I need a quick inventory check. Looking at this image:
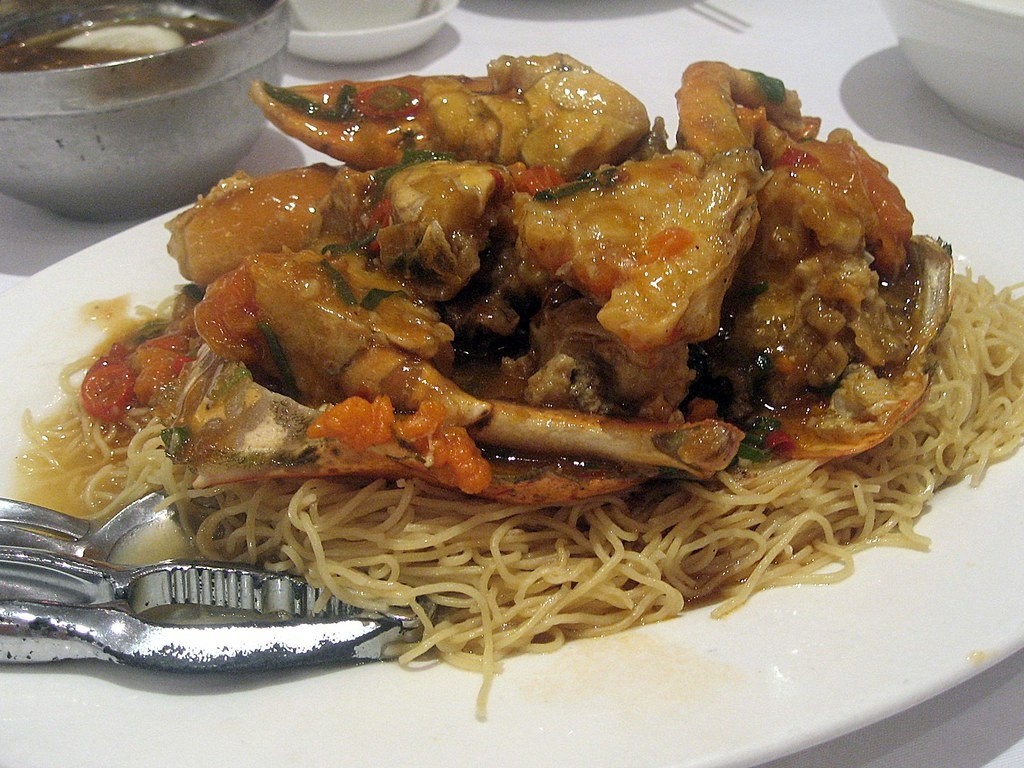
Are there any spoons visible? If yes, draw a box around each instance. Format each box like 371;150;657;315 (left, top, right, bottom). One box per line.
0;489;268;567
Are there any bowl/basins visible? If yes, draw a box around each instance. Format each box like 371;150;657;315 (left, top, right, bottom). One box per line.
0;1;290;223
885;0;1024;147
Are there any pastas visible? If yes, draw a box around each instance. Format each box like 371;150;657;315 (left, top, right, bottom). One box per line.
18;254;1024;719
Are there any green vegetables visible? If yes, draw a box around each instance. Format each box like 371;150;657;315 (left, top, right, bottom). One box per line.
249;67;788;397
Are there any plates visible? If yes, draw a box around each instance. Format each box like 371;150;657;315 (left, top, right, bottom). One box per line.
3;146;1023;767
282;1;459;63
